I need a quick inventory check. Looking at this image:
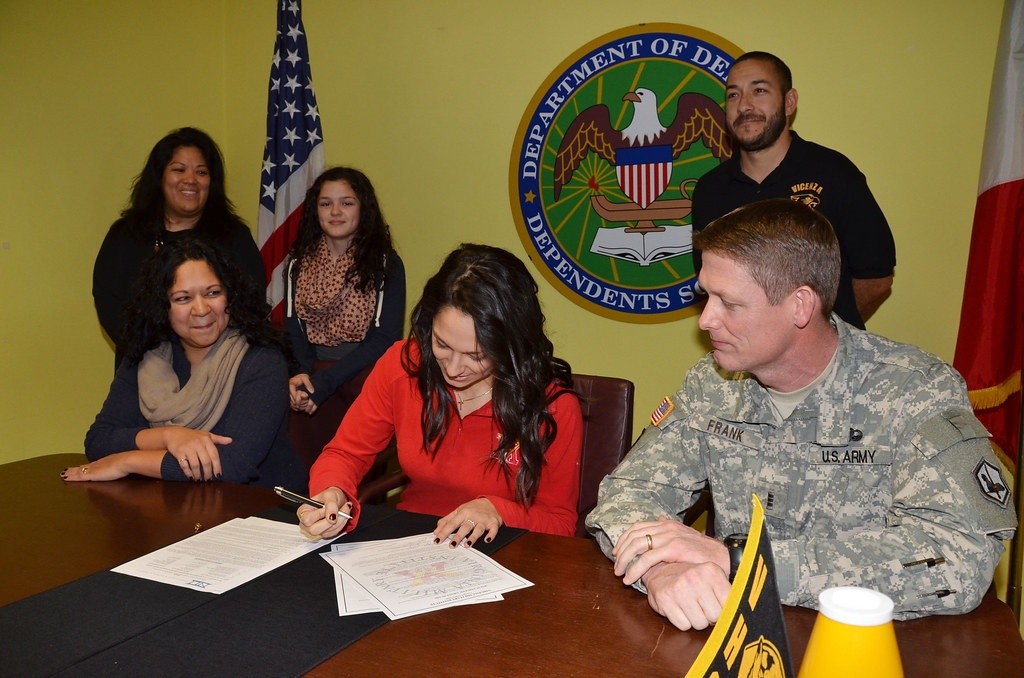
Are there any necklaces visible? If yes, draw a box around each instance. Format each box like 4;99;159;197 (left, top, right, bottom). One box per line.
457;387;493;411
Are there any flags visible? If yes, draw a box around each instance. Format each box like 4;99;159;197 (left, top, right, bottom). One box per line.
257;0;326;332
952;0;1024;470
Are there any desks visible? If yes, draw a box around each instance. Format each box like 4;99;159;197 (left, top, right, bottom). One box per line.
0;451;1024;678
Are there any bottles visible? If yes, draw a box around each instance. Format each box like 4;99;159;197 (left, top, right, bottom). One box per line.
798;586;904;678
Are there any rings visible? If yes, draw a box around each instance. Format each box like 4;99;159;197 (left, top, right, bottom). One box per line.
179;459;187;464
468;520;475;526
299;511;306;518
646;534;652;550
82;467;88;474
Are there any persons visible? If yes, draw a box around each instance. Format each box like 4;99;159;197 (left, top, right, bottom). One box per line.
691;51;897;331
59;237;307;494
282;166;406;415
296;243;583;549
584;199;1019;631
92;127;273;374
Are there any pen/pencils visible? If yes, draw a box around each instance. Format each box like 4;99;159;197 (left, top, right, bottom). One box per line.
916;588;958;599
904;556;945;568
274;485;354;520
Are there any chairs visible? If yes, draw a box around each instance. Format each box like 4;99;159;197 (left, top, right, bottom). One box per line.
558;374;634;537
283;359;411;512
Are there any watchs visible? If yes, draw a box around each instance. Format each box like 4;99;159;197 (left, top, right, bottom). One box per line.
723;533;748;585
